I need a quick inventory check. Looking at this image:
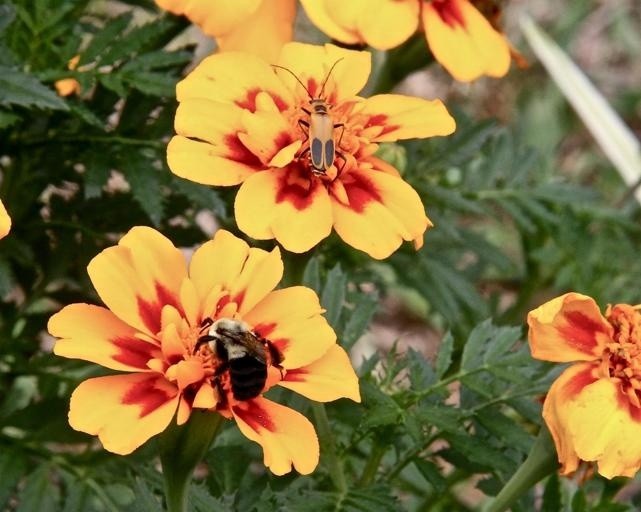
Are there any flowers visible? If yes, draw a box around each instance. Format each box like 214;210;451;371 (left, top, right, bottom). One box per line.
1;0;641;512
